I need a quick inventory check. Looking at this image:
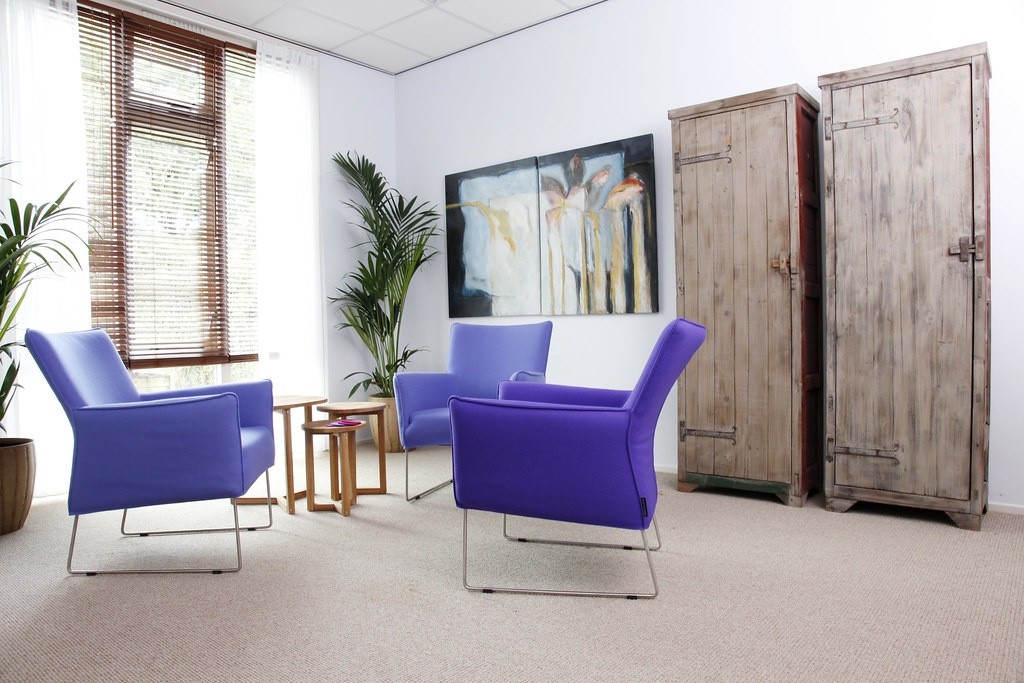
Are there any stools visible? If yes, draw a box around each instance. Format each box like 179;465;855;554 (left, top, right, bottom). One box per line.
302;418;366;517
316;401;385;495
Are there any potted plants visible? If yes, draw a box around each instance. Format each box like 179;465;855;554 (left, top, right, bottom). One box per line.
331;150;449;452
0;161;106;535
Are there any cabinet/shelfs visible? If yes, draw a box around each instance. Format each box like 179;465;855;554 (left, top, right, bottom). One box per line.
816;41;993;531
667;84;822;507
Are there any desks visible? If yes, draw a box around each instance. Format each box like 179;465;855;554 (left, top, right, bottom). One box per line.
230;397;327;515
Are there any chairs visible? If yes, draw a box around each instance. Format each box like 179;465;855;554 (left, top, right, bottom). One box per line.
448;319;706;599
24;327;276;575
393;320;552;501
